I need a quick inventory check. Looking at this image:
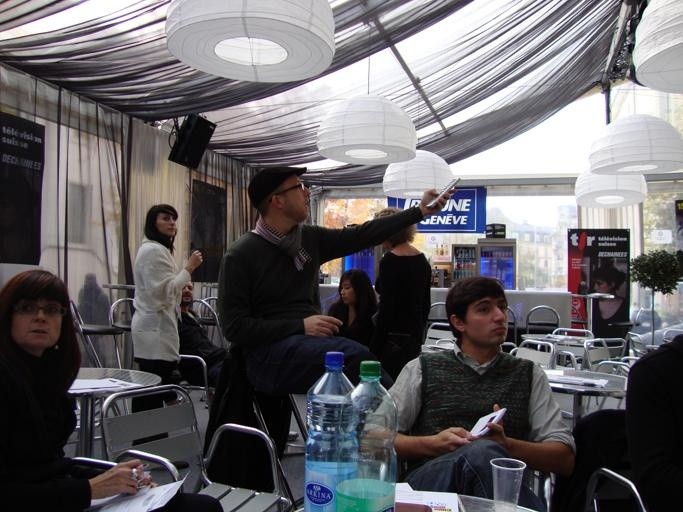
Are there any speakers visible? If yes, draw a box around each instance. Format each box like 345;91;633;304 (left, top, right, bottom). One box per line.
168;113;217;171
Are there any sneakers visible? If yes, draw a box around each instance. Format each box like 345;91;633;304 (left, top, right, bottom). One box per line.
287;431;301;443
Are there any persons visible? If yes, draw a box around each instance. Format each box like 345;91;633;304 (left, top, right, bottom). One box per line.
583;265;627;345
162;281;230;405
0;269;222;511
218;166;458;395
327;268;377;344
373;206;433;380
361;275;577;512
625;334;680;512
130;205;203;444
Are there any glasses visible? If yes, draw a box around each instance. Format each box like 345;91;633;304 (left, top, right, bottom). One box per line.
14;300;70;320
268;182;310;206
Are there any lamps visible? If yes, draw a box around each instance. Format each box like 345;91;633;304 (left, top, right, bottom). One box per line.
383;91;455;199
632;0;683;93
589;83;683;175
317;23;418;165
575;169;647;209
165;0;336;84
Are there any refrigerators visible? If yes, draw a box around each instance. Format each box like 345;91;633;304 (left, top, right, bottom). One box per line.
448;237;518;291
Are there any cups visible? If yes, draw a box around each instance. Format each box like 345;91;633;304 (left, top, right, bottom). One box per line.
488;456;528;512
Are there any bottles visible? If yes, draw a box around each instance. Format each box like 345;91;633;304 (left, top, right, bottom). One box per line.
332;359;400;512
480;247;513;257
430;266;451;289
303;350;362;512
452;248;476;281
435;243;450;256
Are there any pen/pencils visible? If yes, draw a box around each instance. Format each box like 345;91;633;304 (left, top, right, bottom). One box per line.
135;463;150;470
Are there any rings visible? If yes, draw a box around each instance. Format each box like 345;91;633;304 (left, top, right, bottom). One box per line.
132;468;138;478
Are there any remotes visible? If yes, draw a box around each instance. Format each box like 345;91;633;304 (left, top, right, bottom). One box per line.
425;177;461;208
